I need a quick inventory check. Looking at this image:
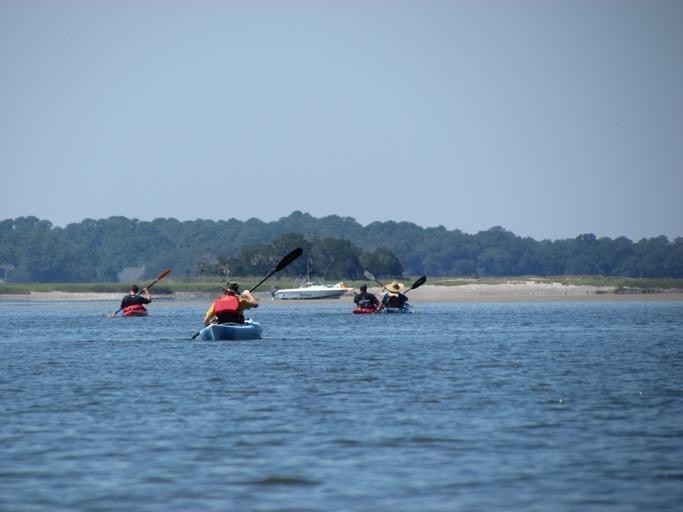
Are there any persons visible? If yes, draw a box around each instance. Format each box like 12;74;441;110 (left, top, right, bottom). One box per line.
119;284;151;310
353;282;380;309
202;279;258;326
375;280;407;311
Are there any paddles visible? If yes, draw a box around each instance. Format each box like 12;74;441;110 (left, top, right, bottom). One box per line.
107;268;171;318
191;247;304;340
363;270;384;287
402;275;426;294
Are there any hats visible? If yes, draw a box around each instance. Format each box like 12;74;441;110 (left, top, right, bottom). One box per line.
386;281;403;292
222;282;240;294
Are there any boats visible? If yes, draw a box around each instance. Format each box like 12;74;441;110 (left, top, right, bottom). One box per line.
197;317;266;342
350;299;379;315
381;300;417;316
120;304;151;317
270;257;354;302
